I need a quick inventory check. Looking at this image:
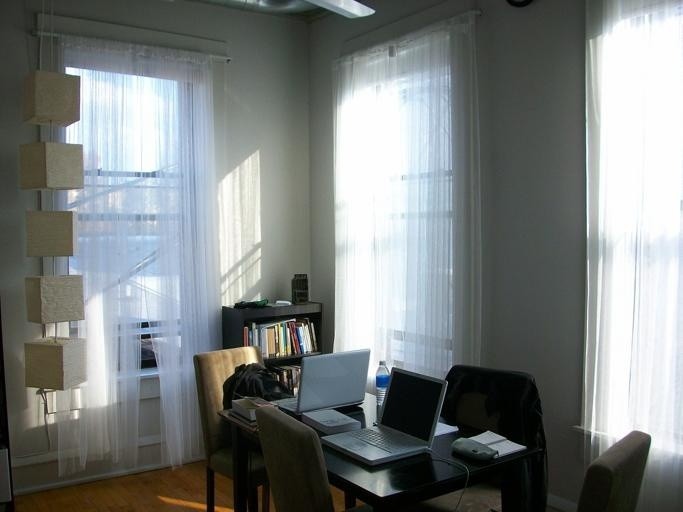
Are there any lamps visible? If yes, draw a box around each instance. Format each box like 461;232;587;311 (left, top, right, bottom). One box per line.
18;0;87;393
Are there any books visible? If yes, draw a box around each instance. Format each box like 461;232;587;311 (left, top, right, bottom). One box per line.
264;364;300;397
469;430;527;457
229;392;278;426
434;421;459;436
244;318;317;359
302;408;361;436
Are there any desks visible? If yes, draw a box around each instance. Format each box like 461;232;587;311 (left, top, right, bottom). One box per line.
219;392;545;510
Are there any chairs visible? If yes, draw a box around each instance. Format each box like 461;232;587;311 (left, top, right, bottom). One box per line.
577;430;652;512
441;365;547;509
192;345;271;511
256;406;336;512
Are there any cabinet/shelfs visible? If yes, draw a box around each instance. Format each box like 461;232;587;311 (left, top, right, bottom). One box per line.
221;302;323;397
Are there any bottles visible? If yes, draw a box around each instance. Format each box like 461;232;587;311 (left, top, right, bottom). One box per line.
375;359;391;407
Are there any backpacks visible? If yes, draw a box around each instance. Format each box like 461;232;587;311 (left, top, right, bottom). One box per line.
222;363;295;408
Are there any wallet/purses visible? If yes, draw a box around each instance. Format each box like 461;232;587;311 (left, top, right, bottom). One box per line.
451;438;499;460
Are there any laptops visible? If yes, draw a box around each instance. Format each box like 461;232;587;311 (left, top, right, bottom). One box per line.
271;348;370;415
319;366;448;467
